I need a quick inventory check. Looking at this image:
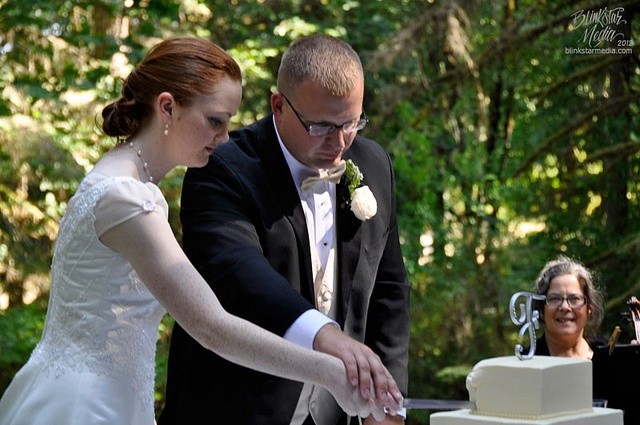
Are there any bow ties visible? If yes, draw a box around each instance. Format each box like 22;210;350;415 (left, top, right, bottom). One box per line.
300;160;346;196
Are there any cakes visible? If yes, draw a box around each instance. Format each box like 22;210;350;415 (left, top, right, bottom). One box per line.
465;291;593;417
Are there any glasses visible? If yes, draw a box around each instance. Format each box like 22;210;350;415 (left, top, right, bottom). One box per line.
280;89;369;136
544;292;587;309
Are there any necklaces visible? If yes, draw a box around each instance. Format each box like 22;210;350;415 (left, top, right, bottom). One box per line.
121;138;154;182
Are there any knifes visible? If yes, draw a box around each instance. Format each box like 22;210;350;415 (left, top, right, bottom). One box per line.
398;398;476;409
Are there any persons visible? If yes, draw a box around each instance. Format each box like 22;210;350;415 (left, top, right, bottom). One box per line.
0;37;401;424
157;35;411;425
518;257;609;409
